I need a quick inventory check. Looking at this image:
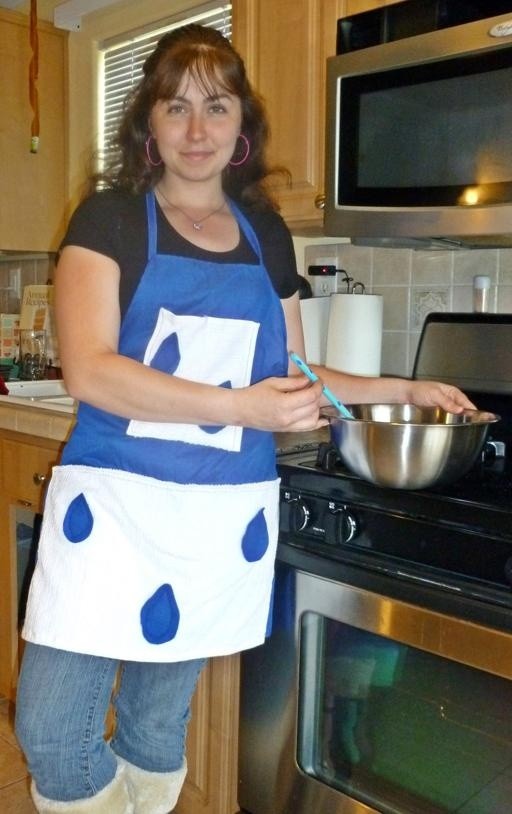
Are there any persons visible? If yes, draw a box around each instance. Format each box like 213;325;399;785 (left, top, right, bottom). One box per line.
15;23;477;814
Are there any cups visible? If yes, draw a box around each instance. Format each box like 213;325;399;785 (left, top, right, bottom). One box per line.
18;328;47;380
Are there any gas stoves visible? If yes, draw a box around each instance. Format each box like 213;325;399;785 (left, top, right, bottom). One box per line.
274;429;510;514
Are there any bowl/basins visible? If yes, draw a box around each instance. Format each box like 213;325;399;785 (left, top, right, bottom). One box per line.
319;403;503;491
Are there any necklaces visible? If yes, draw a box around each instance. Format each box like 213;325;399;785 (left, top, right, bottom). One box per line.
156;185;227;231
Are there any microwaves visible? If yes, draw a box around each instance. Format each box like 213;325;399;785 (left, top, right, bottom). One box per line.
325;12;512;239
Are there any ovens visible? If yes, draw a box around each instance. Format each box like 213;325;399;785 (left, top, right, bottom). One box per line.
235;485;512;813
